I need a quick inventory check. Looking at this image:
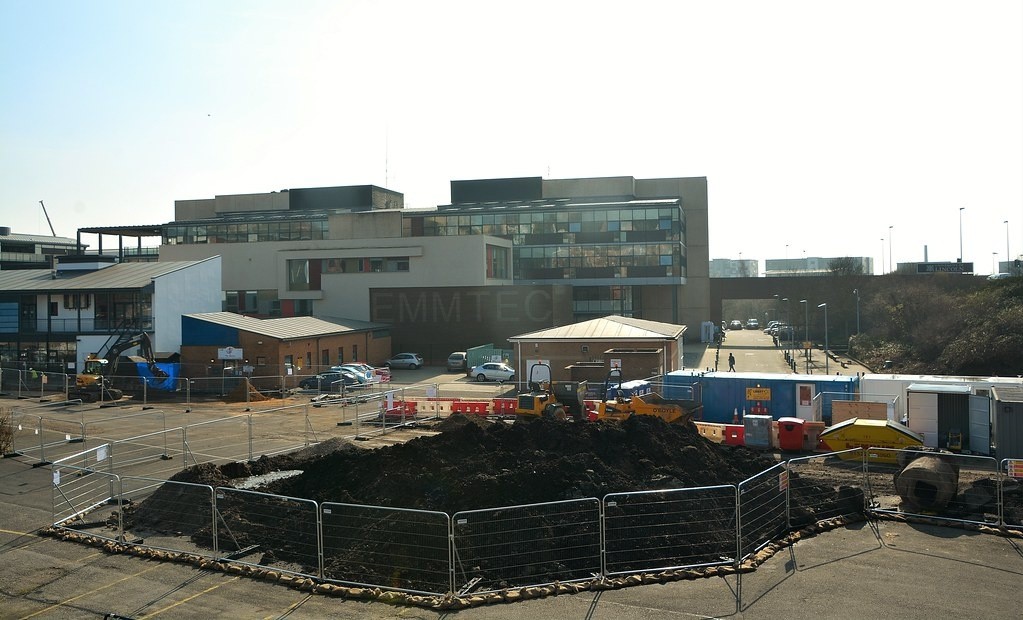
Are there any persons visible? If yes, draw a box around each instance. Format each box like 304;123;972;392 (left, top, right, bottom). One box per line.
29;368;38;384
727;353;735;372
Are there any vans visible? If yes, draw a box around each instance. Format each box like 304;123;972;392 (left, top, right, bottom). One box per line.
987;272;1011;279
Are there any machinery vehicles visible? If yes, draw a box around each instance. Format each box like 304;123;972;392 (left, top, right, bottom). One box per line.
65;329;171;403
514;359;562;420
580;362;699;445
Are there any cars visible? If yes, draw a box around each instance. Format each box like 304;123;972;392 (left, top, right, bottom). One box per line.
721;320;728;337
730;320;742;330
298;370;359;392
763;320;788;336
329;362;392;386
447;352;467;372
746;318;760;330
470;361;516;383
382;353;424;370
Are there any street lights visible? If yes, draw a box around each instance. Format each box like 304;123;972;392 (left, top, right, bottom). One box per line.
880;238;884;275
852;289;859;334
889;225;894;274
992;252;998;275
1004;220;1010;273
800;299;809;357
818;302;829;375
959;207;965;261
782;297;790;353
